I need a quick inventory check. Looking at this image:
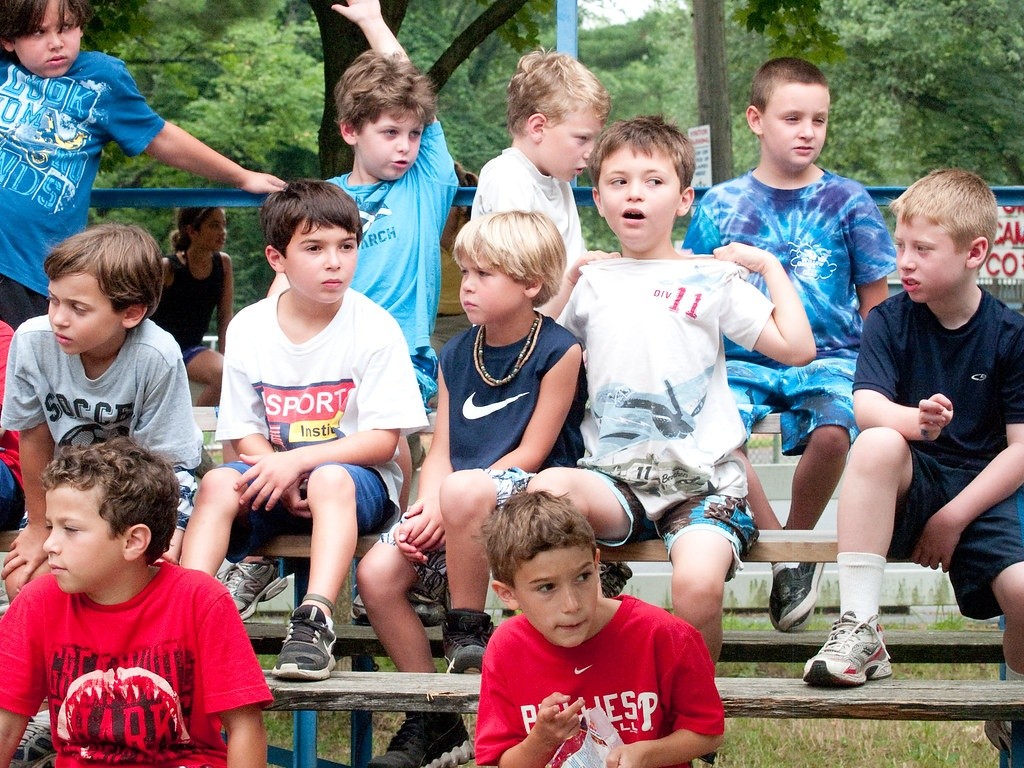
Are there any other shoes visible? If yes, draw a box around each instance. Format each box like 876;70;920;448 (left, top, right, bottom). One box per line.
598;558;631;598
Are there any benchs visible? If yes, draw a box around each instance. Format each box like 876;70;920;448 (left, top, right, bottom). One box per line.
193;403;1022;766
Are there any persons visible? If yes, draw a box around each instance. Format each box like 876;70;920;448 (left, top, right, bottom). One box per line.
474;491;724;768
355;211;588;768
470;51;611;326
0;222;204;604
0;0;285;332
219;0;459;619
180;179;430;680
544;116;817;665
0;435;275;768
683;57;896;631
0;320;25;532
148;206;234;480
804;169;1024;768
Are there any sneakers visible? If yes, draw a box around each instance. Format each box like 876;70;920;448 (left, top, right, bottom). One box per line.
365;710;475;768
803;610;893;684
217;559;288;622
768;561;825;632
350;583;370;627
982;719;1013;768
442;608;494;674
9;716;57;768
272;606;336;679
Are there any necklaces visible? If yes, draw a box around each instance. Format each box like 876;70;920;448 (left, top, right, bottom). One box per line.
474;313;543;386
